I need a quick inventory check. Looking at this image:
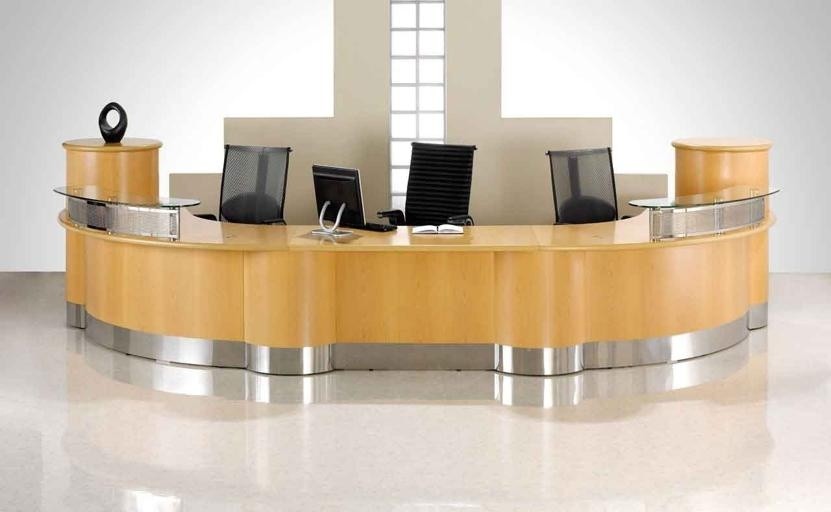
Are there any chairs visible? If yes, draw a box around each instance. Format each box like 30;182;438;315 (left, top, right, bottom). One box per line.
202;142;292;230
545;145;621;225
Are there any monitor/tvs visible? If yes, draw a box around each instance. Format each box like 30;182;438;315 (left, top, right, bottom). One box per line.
312;165;367;237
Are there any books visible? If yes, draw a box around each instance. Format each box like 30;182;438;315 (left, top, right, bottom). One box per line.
411;224;465;234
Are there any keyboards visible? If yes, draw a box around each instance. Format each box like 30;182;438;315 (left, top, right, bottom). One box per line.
360;222;397;232
